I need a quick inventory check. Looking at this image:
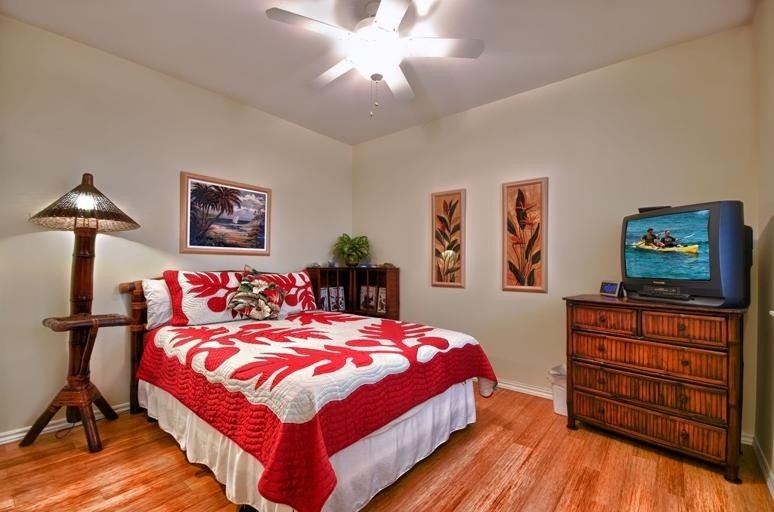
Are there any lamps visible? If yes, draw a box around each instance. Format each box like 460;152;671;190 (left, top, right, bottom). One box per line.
18;173;141;454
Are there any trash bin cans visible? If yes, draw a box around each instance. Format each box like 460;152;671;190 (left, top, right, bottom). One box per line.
547;363;568;417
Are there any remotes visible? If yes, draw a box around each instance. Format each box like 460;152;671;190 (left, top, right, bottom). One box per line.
638;205;670;213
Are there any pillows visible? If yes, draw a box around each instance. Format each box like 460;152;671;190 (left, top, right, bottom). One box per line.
140;263;319;330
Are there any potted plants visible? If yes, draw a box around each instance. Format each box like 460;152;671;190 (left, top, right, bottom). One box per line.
335;233;371;266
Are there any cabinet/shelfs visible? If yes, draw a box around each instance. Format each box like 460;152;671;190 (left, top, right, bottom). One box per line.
561;291;748;485
305;266;400;321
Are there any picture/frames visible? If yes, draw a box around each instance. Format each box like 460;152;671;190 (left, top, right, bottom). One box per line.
501;177;549;294
179;171;273;257
429;188;466;288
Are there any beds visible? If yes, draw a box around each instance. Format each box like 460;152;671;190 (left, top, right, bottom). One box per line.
118;273;478;512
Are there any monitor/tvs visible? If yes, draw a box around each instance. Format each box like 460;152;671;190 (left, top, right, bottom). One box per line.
620;199;753;309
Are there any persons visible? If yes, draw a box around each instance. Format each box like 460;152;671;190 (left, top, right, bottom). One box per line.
662;231;679;248
640;228;663;250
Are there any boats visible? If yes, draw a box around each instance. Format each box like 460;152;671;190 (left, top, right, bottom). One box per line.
632;241;699;254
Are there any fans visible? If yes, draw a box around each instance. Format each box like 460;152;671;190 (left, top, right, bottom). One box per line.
265;0;485;104
